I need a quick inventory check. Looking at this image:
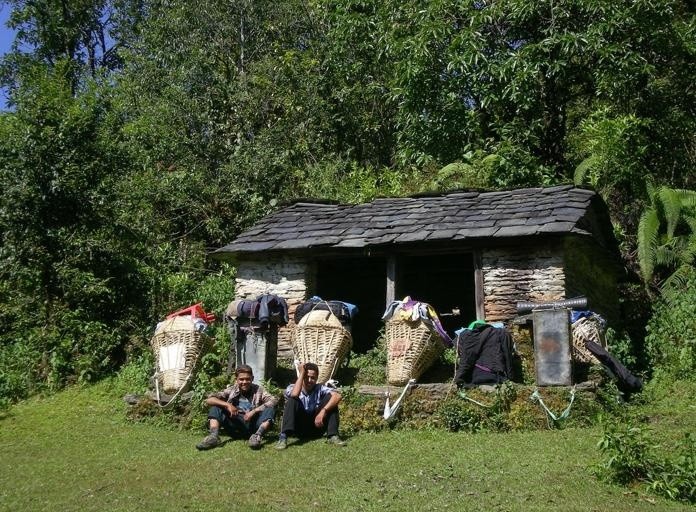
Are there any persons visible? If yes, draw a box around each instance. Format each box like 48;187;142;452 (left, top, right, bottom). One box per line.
195;364;279;450
274;363;348;450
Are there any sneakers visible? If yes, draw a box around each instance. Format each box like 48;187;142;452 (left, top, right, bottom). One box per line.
249;434;260;448
277;439;287;450
327;436;346;446
195;435;221;449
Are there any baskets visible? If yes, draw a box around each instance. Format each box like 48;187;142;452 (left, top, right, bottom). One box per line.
150;329;215;396
289;323;353;386
569;315;608;365
385;320;446;387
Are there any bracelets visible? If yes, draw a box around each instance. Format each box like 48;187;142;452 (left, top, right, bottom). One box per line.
251;410;255;415
322;407;327;412
